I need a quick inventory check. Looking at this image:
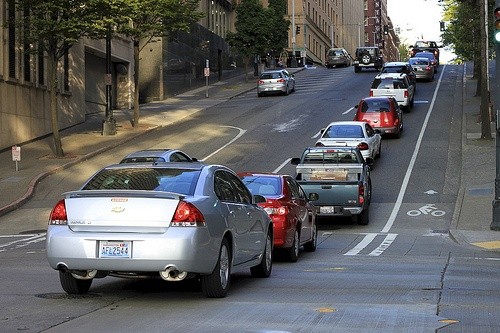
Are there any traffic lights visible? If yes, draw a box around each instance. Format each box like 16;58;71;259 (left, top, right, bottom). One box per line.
493;5;500;47
439;22;445;30
377;25;389;41
295;26;300;34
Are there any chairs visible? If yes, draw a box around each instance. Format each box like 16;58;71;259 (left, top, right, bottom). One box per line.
258;185;276;195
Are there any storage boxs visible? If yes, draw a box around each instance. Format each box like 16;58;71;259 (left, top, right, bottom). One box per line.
311;170;348;182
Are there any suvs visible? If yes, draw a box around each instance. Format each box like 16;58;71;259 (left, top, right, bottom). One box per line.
407;41;443;81
369;61;417;111
352;47;385;73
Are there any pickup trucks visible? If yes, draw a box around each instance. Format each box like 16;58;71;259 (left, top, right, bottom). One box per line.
291;147;373;225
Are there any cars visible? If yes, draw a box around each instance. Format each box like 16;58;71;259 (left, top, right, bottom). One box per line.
355;96;405;140
256;68;295;96
314;121;383;171
48;162;275;297
234;171;320;263
324;47;351;69
121;150;201;163
